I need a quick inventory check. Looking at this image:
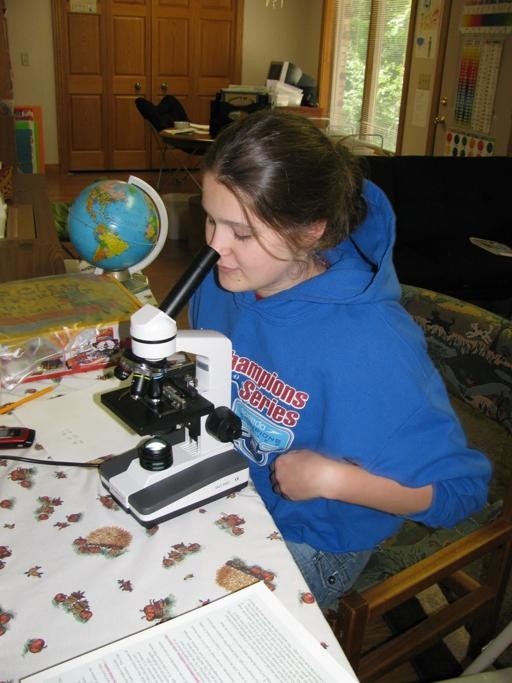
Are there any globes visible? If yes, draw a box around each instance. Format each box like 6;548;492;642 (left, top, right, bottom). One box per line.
66;174;169;283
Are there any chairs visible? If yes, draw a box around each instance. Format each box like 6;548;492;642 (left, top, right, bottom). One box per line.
327;283;510;681
136;96;213;192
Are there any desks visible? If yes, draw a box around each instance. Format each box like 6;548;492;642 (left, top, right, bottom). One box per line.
0;273;361;683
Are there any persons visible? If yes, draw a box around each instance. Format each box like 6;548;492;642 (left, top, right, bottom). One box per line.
186;110;492;608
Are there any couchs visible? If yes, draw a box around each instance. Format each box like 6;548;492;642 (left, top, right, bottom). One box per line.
356;157;508;285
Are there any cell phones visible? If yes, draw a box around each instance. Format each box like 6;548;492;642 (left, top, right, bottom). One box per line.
0;427;35;449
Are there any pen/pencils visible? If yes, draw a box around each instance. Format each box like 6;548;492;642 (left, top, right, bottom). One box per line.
0;384;60;415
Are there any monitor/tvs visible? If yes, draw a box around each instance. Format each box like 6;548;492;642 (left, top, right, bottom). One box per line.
264;60;315;88
